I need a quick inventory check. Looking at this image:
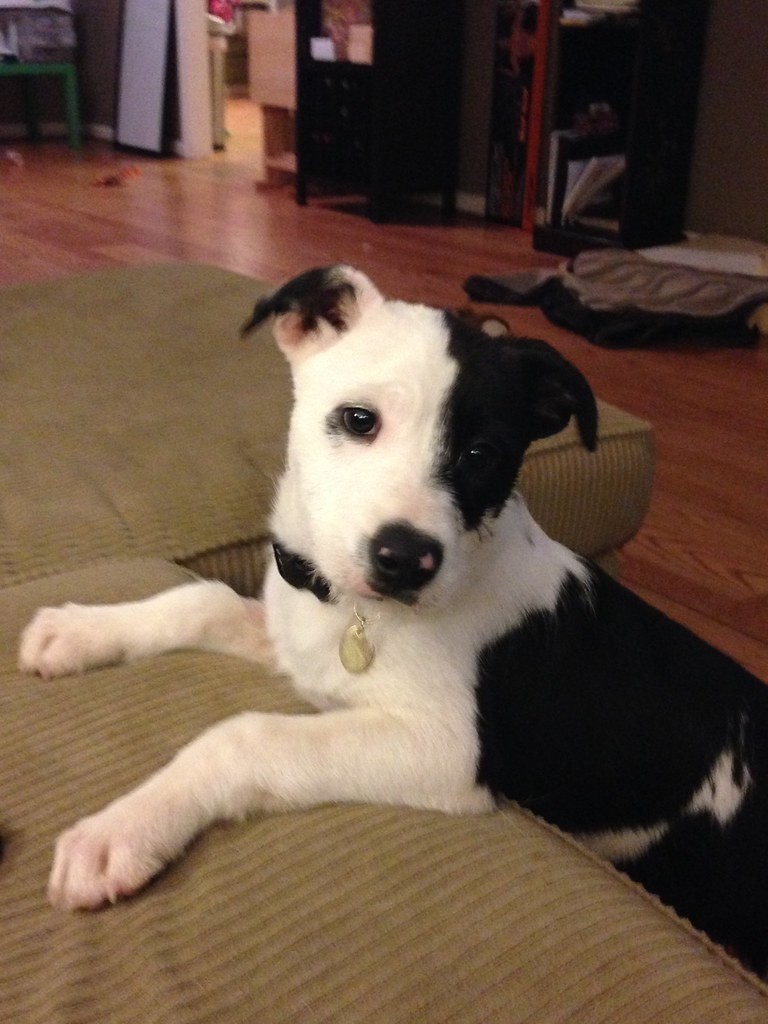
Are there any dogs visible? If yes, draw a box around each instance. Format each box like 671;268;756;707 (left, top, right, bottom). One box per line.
13;263;768;993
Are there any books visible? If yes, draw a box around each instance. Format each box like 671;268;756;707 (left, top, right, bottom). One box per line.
547;129;623;233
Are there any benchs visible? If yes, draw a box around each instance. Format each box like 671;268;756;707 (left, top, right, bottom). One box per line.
0;253;768;1024
